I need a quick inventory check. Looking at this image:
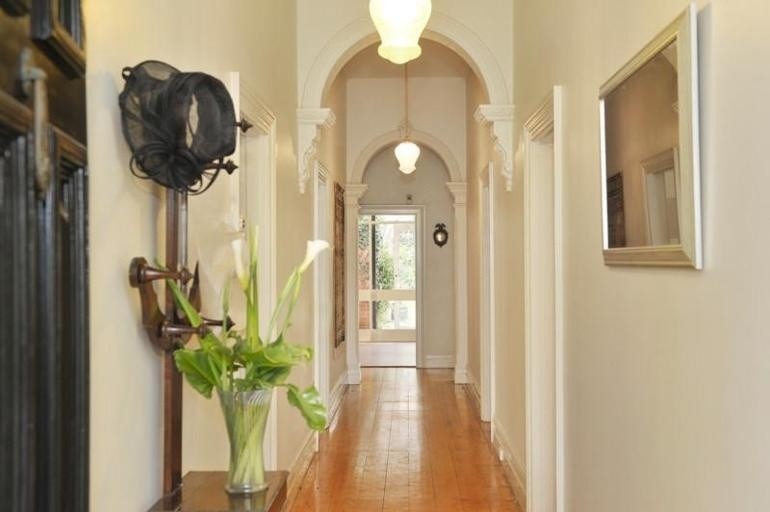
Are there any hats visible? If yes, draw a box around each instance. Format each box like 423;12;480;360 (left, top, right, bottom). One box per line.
118;59;237;197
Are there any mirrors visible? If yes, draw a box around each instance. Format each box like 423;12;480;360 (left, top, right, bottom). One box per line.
597;2;700;269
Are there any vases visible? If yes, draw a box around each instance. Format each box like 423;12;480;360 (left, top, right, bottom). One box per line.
215;389;270;493
148;225;334;431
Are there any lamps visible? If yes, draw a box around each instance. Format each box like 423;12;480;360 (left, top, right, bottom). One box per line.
368;0;433;65
394;62;421;174
